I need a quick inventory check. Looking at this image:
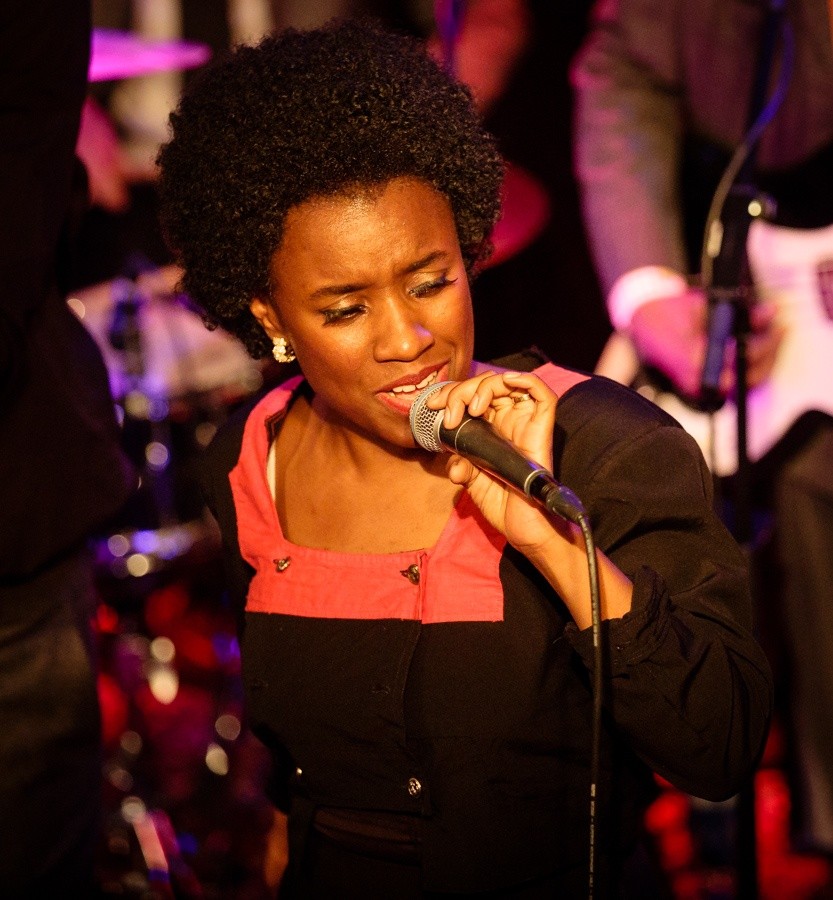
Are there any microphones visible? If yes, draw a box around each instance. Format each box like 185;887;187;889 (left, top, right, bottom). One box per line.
407;380;590;524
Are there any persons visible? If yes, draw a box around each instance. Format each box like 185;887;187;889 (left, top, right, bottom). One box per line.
154;21;769;900
572;1;833;812
